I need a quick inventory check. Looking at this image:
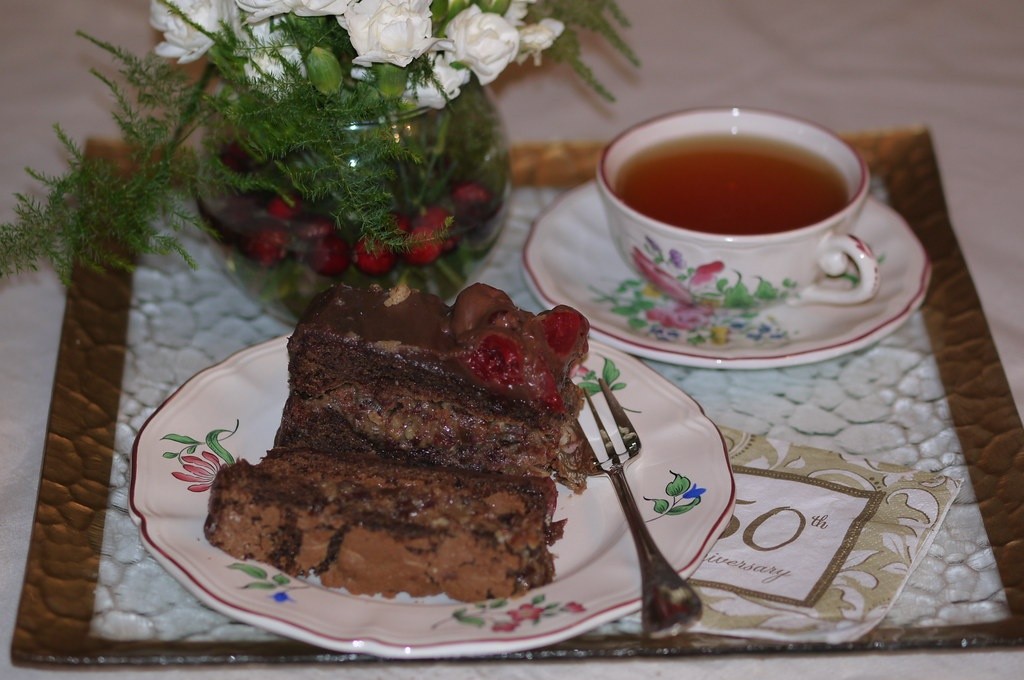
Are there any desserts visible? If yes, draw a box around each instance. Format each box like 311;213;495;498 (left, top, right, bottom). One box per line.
204;282;593;603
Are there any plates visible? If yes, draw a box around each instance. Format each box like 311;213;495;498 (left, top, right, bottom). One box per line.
129;328;737;661
522;173;934;371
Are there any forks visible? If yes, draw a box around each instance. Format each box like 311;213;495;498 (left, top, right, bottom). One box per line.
568;377;704;639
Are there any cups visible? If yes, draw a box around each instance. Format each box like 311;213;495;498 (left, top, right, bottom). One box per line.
595;105;878;306
